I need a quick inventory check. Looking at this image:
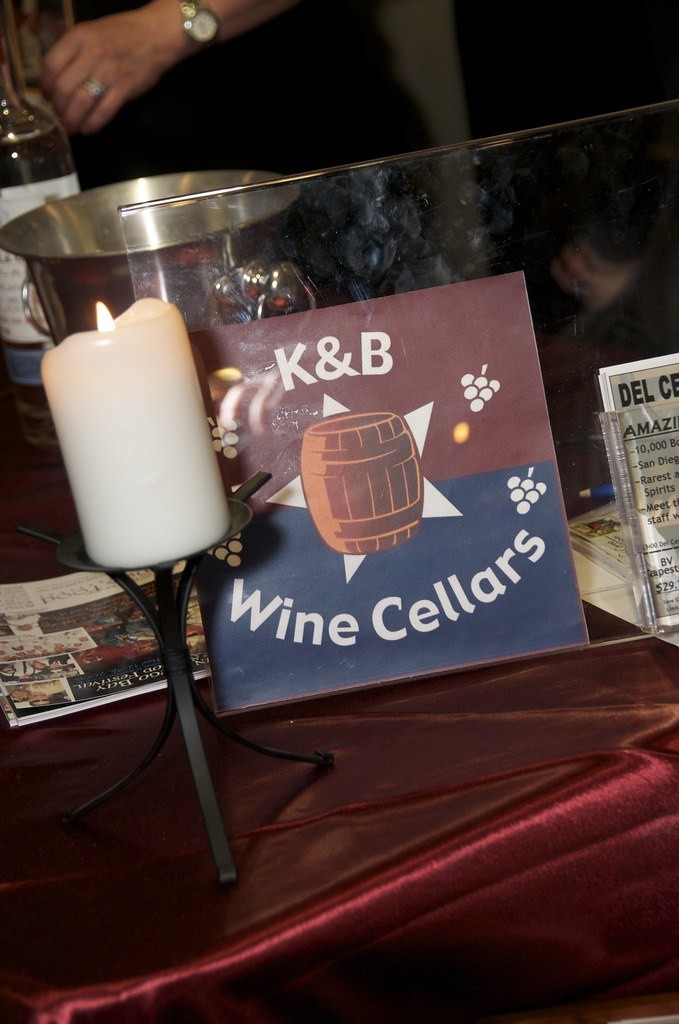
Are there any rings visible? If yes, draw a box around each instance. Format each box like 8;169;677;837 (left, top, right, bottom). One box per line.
83;78;104;98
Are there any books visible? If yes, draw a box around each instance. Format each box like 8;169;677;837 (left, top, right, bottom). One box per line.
570;500;630;583
0;560;210;728
196;271;589;716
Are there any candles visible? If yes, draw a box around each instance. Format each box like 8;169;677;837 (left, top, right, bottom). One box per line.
40;296;231;570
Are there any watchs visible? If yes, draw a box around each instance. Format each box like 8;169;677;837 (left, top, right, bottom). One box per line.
180;0;217;51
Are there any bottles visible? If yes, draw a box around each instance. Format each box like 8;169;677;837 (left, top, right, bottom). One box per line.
0;18;85;451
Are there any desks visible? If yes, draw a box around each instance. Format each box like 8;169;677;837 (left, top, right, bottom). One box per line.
0;456;679;1024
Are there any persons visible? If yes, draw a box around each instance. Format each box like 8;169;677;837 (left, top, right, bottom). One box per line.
20;0;51;74
0;0;679;353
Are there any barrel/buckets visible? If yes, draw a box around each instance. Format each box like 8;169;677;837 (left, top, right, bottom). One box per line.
0;168;318;361
